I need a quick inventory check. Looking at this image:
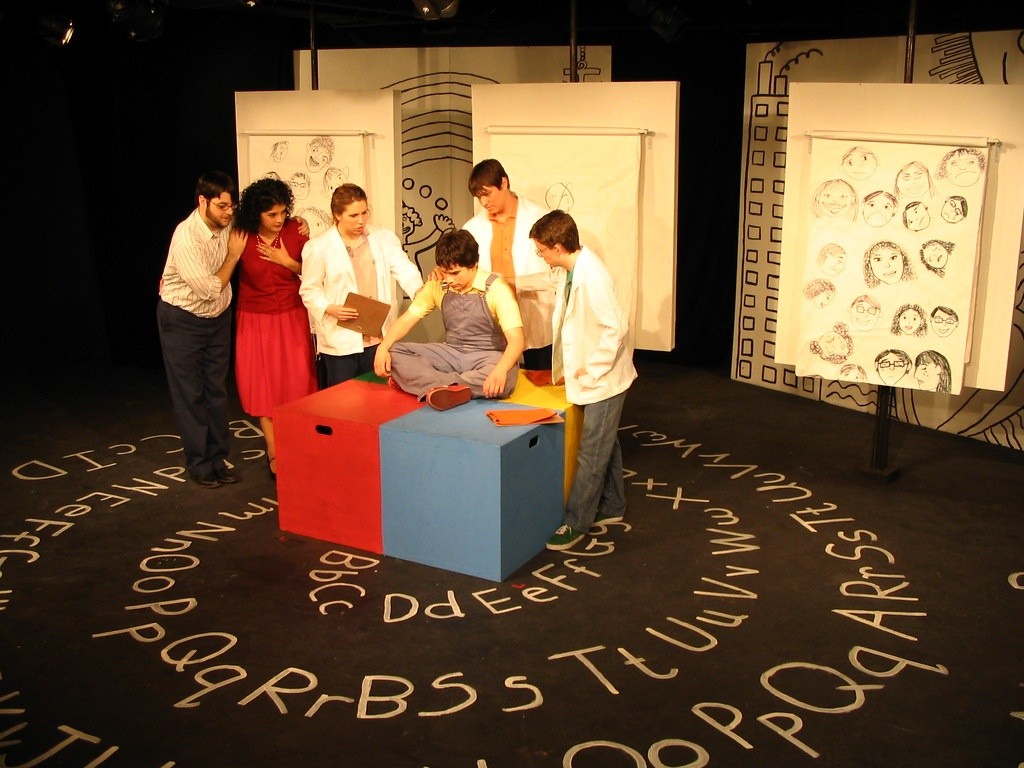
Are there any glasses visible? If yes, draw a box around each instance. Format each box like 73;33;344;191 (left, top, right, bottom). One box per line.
535;247;549;258
206;198;239;211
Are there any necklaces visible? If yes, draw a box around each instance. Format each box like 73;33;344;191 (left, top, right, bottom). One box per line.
257;232;279;255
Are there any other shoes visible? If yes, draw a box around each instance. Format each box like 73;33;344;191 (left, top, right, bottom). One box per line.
220;474;239;483
265;449;277;482
546;525;587;551
590;509;624;527
426;385;473;412
195;477;222;488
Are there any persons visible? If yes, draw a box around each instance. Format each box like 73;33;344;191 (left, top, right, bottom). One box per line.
160;179;318;480
374;229;524;410
157;172;249;487
299;184;425;388
427;160;558;371
506;209;637;550
